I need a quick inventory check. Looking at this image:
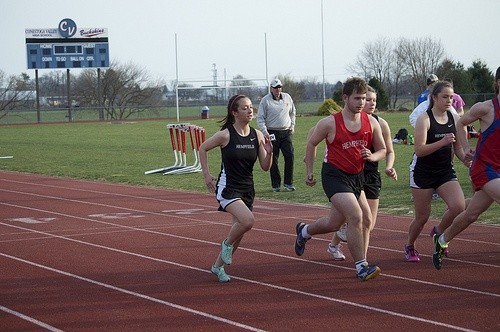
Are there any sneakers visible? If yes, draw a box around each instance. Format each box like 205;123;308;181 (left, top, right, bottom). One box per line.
336;223;348;242
357;264;381;281
293;222;311;256
430;225;449;256
211;263;230;283
328;244;345;261
220;239;234;265
404;244;420;262
432;234;449;270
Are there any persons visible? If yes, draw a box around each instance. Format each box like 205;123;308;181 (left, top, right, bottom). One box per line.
199;95;273;282
408;81;460;200
405;80;472;262
294;78;387;281
418;74;438;105
303;84;398;261
256;79;296;193
433;66;500;270
444;78;468;145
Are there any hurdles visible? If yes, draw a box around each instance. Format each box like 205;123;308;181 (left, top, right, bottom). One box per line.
146;124;206;175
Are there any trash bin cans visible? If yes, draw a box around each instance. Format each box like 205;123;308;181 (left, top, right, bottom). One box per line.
201;106;209;119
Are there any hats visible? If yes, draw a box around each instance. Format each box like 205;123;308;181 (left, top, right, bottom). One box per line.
271;79;283;88
427;74;439;84
